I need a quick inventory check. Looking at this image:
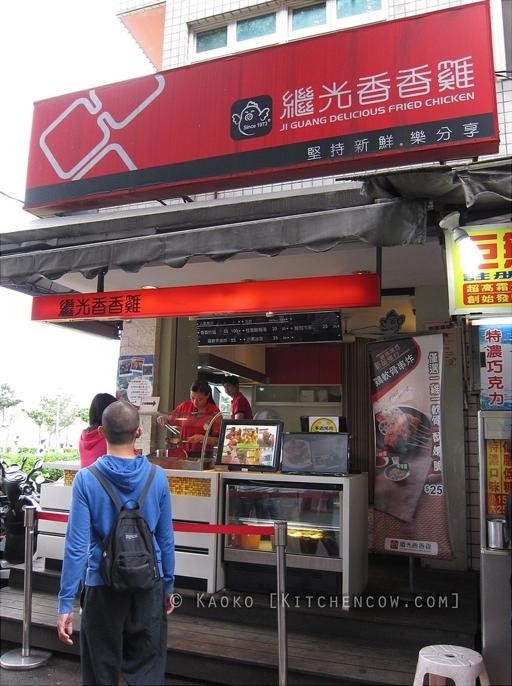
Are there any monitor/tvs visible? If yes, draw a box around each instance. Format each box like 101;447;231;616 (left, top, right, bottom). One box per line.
279;431;352;477
214;418;285;473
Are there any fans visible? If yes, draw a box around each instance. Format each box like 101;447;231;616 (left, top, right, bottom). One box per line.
341;315;384;343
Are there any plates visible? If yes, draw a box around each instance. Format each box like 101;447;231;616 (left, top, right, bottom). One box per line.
375;407;431;454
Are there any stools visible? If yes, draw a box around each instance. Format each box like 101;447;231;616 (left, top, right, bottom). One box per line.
413;644;491;686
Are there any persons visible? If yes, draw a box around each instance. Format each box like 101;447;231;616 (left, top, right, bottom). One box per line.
58;393;177;686
223;376;256;420
157;379;223;457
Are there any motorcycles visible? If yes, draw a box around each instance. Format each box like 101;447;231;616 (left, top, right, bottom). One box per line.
0;458;45;582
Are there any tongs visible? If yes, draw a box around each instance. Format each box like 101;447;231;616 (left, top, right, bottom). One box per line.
163;423;180;435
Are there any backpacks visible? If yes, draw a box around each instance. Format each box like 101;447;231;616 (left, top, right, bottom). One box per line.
86;464;160;591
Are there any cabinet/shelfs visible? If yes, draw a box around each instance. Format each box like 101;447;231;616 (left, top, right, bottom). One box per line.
216;471;369;612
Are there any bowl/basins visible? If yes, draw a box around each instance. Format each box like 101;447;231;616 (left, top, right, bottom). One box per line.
375;456;389;469
385;464;411;482
